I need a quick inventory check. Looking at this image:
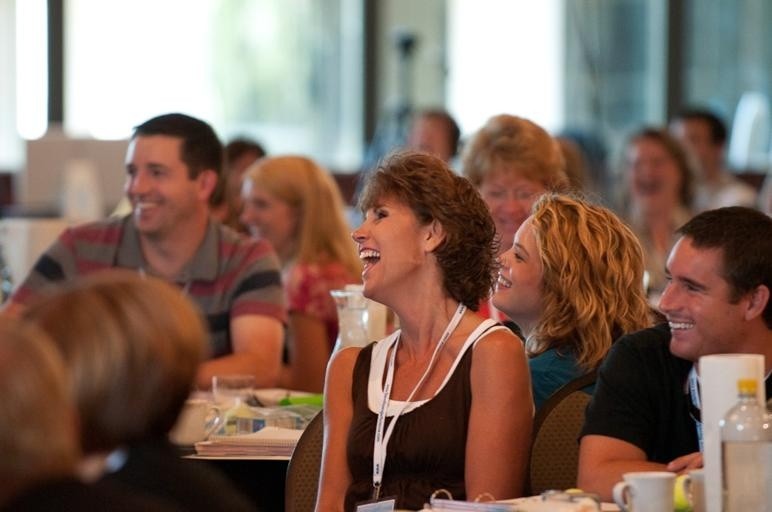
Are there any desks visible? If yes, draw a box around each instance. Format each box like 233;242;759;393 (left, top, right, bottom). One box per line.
176;388;323;465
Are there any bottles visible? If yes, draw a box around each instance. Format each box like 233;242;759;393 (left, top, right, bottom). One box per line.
330;290;372;355
718;374;771;512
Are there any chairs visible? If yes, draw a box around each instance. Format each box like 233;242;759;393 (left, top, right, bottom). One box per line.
281;404;327;509
527;372;600;495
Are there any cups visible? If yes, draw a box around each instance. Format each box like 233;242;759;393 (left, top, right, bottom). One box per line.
682;468;705;510
168;398;222;444
214;374;256;428
613;471;675;512
